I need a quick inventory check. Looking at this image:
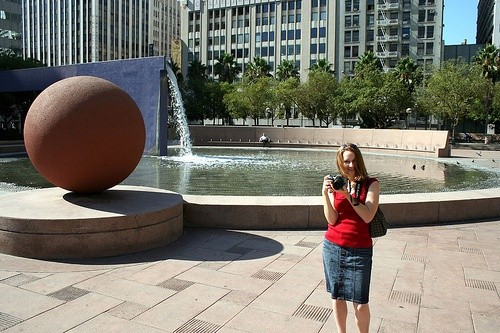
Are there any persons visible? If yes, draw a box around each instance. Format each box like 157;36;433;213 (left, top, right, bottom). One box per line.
322;143;379;332
260;132;268;146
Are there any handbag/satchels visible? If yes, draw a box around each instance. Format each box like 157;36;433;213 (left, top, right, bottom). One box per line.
369;206;387;237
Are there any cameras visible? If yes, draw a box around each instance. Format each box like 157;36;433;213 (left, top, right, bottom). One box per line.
329;174;345;190
